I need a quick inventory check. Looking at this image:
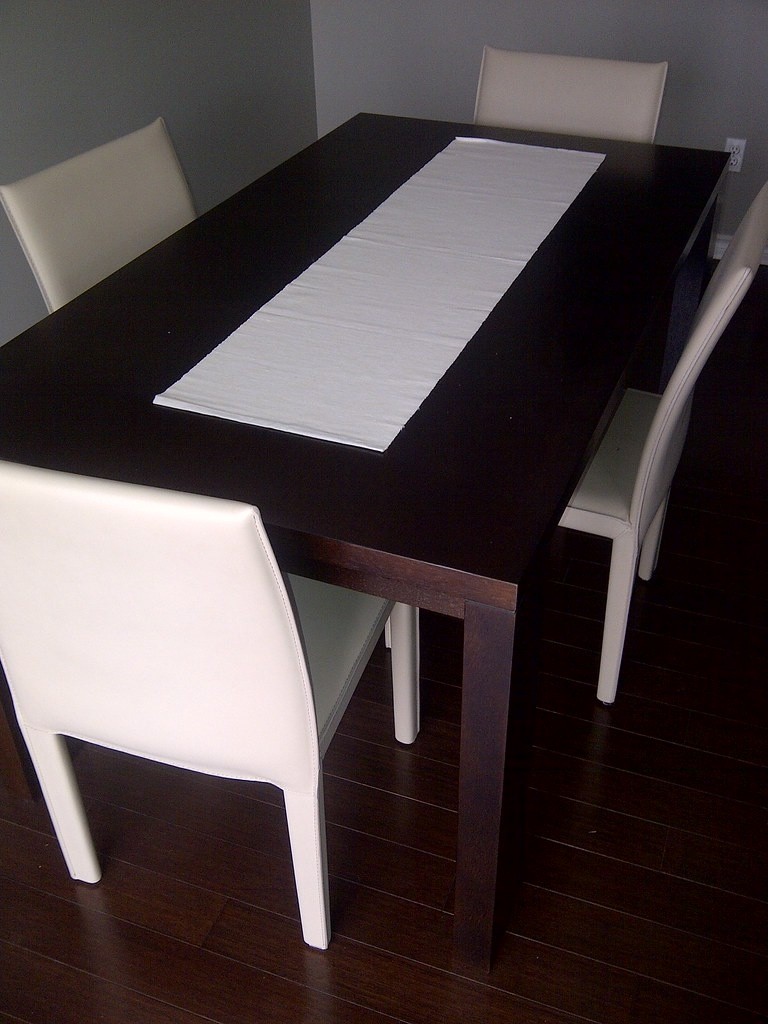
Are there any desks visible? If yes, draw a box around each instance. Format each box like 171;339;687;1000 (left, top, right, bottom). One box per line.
0;113;733;970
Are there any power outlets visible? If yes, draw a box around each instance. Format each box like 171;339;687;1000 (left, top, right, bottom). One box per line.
724;137;747;171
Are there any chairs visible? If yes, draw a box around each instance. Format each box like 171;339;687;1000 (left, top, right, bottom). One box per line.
474;46;671;144
557;182;768;708
0;458;423;951
0;116;198;316
